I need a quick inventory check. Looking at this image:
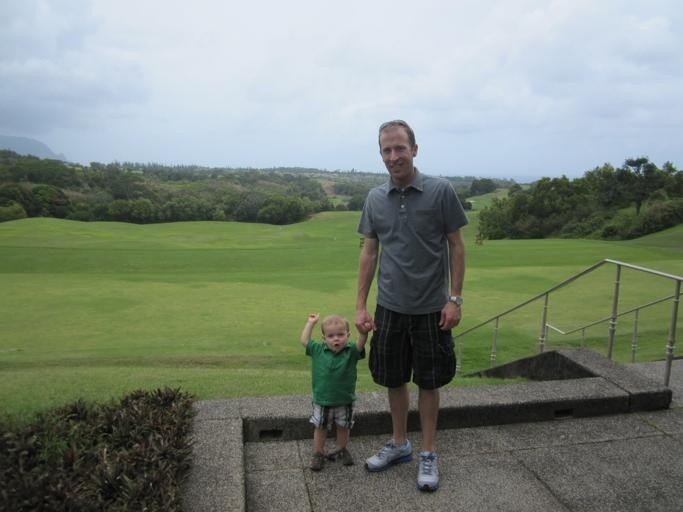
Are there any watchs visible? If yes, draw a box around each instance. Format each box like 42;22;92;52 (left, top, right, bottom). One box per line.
447;295;464;307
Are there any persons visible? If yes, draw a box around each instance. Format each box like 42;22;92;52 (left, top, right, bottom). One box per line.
353;119;469;490
299;313;371;472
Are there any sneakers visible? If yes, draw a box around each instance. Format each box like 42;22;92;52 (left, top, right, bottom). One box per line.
310;451;325;471
337;448;354;466
364;438;414;472
417;451;439;492
